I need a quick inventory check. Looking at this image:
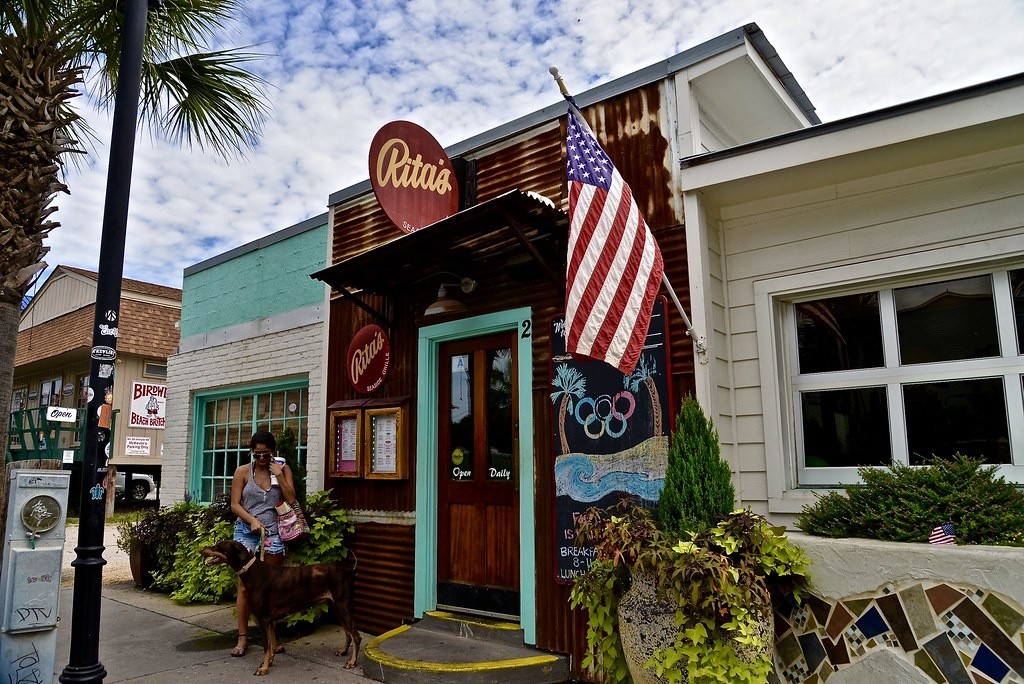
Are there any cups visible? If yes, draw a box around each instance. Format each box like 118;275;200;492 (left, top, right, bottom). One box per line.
270;457;286;486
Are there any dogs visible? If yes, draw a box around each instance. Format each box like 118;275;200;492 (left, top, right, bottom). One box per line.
199;539;361;675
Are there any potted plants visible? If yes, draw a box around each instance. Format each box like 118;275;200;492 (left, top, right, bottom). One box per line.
563;499;828;684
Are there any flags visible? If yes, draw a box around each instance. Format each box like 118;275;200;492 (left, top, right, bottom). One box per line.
563;98;664;374
928;522;958;545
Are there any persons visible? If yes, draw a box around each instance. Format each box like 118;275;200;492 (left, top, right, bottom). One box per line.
229;429;296;657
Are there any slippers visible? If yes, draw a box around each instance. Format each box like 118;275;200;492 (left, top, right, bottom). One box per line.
231;645;247;656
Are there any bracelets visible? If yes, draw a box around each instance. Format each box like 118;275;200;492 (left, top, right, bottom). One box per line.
276;473;283;477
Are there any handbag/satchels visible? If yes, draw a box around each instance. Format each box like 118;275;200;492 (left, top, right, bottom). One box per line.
275;499;311;544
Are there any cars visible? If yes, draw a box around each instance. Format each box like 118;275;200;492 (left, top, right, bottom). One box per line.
97;469;156;500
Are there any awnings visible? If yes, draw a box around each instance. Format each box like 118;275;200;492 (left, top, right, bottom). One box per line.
307;189;570;327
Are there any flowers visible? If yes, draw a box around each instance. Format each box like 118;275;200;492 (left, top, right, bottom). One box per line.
112;506;173;554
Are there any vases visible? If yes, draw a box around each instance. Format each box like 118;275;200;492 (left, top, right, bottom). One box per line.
129;541;159;586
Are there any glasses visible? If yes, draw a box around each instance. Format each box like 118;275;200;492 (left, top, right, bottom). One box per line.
252;449;272;461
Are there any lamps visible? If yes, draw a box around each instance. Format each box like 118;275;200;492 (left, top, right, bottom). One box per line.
424;277;478;318
